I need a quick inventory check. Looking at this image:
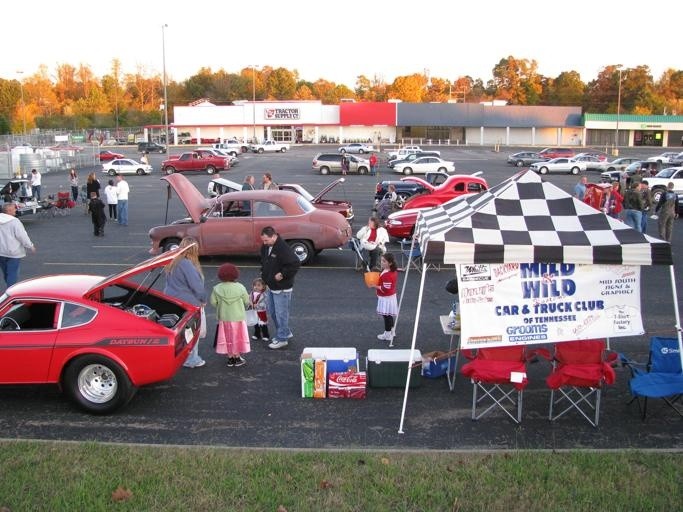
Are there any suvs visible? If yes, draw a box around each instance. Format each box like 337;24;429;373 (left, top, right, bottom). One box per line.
137;141;166;154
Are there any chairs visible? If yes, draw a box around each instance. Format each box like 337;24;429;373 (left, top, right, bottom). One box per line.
546;334;611;426
38;191;76;218
461;344;529;425
425;261;439;272
397;240;422;271
351;239;384;272
629;335;682;421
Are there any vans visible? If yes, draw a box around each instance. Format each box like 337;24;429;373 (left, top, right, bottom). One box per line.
311;151;376;175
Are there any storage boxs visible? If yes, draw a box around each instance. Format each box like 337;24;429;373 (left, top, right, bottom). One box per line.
421;350;456;379
300;346;358;372
364;348;423;388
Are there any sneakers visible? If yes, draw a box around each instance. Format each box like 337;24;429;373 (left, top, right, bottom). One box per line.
195;359;206;367
391;327;396;337
376;332;393;340
252;332;294;349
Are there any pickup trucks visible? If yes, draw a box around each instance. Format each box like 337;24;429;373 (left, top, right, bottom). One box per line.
251;137;291;155
160;138;249;175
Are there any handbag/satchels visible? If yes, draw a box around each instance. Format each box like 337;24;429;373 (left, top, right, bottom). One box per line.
245;303;259;327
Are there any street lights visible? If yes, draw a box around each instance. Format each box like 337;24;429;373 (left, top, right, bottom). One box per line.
161;21;172;146
14;67;29;135
611;63;628;155
248;63;258;139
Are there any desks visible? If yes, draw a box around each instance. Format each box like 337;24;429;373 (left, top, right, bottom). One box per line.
439;314;461;392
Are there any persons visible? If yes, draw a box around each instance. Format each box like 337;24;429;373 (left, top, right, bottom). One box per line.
573;167;680;244
260;173;279;190
341;153;399;341
681;135;683;146
242;175;255;210
0;168;130;289
207;174;222;198
164;226;301;369
139;154;148;164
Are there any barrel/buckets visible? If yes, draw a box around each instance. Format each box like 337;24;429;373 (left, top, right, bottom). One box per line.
364;272;380;288
245;308;258;327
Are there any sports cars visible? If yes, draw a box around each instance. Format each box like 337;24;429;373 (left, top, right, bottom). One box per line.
93;150;124;160
10;142;43;152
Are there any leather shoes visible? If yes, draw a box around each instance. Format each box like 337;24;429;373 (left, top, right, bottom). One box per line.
235;358;247;367
227;357;234;367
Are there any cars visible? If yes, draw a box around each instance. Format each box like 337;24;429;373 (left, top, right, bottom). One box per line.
148;171;352;264
101;157;152;175
336;142;374;155
373;144;490;243
0;242;206;415
47;141;84;154
505;143;683;212
208;175;355;231
